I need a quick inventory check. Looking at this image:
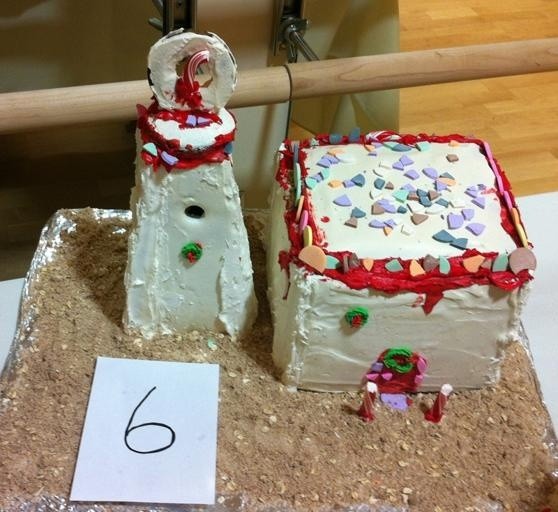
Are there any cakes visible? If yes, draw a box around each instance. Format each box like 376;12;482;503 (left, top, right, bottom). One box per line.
121;27;258;336
262;128;537;394
